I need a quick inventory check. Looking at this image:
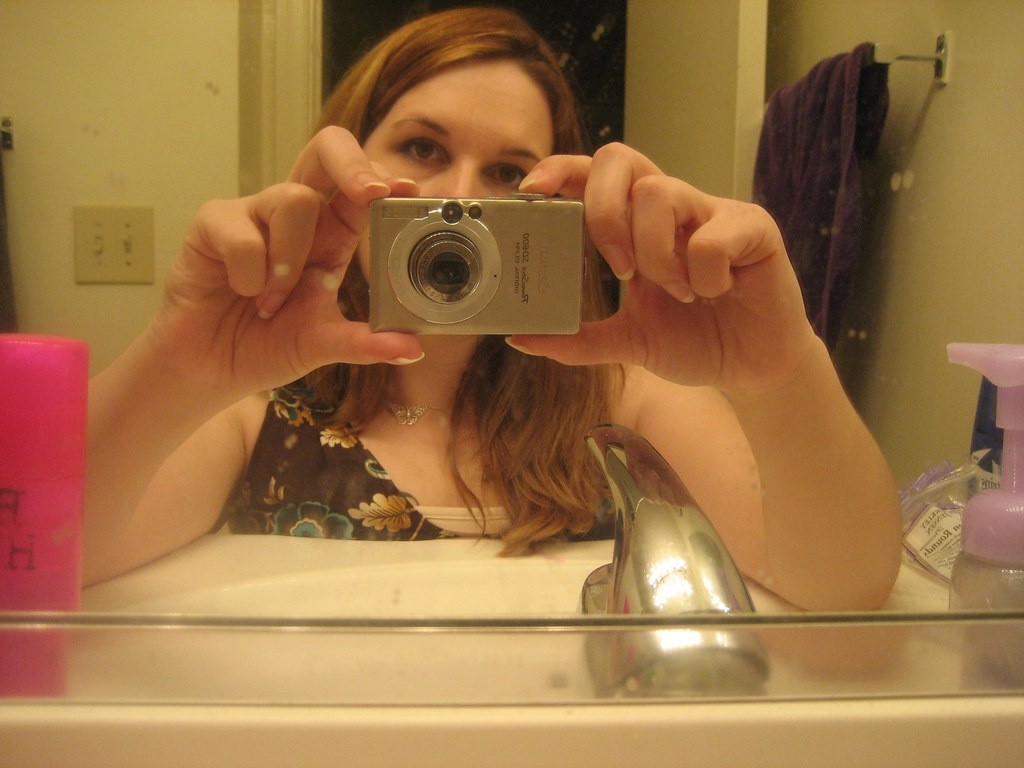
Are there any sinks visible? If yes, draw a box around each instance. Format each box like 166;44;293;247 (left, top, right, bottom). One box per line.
68;560;960;705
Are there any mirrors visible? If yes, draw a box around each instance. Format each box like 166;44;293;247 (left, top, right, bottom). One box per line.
0;0;1024;700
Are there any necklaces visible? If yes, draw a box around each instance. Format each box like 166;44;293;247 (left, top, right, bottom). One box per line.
395;396;447;426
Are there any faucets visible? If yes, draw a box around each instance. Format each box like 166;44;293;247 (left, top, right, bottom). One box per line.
580;425;770;698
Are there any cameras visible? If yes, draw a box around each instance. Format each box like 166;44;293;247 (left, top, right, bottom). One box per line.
368;191;584;334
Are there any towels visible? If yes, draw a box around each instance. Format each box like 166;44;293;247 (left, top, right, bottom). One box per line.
753;41;889;411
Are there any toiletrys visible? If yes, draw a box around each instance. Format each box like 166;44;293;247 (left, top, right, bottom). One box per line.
946;341;1024;692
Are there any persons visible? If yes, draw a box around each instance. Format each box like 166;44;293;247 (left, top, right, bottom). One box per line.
82;7;903;610
